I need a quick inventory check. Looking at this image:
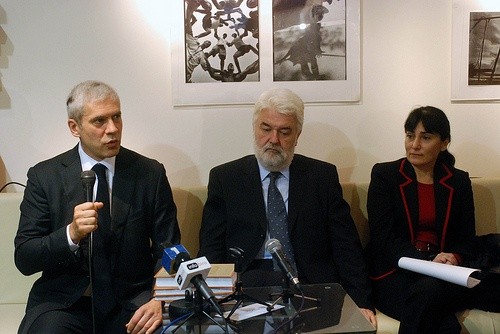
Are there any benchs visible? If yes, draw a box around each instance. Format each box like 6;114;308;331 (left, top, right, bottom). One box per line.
0;176;500;334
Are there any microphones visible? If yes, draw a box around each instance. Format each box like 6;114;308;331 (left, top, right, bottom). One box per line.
265;238;303;294
159;240;223;316
81;170;96;233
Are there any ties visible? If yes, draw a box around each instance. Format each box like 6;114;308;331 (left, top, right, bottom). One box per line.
266;172;299;283
91;162;117;315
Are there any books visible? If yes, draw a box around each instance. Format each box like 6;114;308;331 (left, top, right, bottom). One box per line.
154;263;238;307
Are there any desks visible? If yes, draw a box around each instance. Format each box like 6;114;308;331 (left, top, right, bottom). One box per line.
142;282;378;334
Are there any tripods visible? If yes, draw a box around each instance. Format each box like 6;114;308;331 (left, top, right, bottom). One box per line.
218;246;274;321
172;286;226;334
267;272;322;311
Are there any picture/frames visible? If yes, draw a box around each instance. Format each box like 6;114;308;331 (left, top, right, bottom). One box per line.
450;0;500;102
169;0;362;108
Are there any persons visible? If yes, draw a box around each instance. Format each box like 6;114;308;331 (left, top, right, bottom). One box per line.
196;87;378;328
14;79;181;334
365;105;500;334
